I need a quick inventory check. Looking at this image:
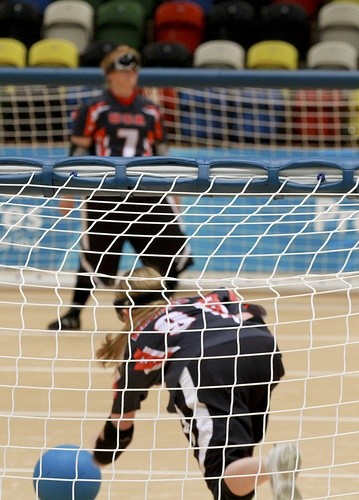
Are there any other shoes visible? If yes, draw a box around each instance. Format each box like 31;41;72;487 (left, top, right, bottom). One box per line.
47;308;80;331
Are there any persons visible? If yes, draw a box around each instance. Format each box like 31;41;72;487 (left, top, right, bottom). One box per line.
37;43;202;335
88;263;306;500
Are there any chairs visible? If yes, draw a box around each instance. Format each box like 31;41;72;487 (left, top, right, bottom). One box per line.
1;0;359;148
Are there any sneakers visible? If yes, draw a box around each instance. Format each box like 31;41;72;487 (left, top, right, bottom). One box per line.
267;443;303;500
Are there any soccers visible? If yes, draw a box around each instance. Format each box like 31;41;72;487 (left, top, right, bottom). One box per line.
32;444;101;500
